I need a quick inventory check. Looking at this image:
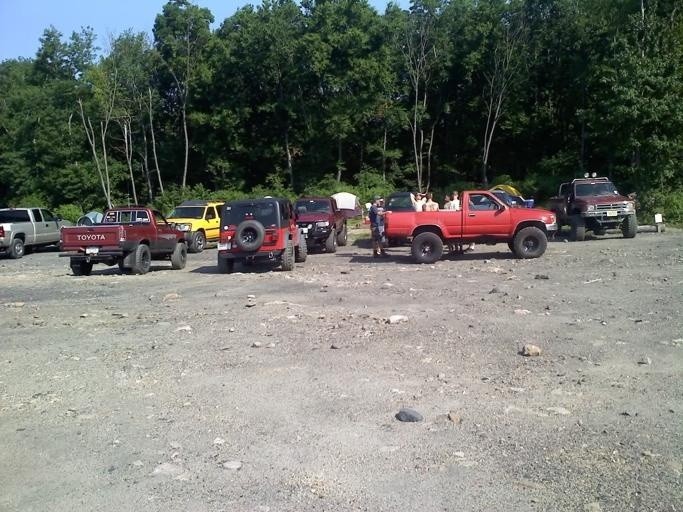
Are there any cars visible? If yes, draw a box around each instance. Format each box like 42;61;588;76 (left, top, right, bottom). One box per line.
373;189;417;215
466;190;536;211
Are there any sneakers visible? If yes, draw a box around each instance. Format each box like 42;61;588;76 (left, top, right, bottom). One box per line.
381;253;391;256
374;254;384;258
464;247;474;252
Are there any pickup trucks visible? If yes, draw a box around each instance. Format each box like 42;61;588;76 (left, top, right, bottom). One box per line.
379;189;564;263
0;207;74;259
54;204;191;277
544;170;641;242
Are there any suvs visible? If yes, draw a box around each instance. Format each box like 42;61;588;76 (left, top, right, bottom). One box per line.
286;194;349;257
214;196;309;273
157;195;226;253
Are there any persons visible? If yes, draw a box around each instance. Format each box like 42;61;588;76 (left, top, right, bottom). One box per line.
368;189;476;259
